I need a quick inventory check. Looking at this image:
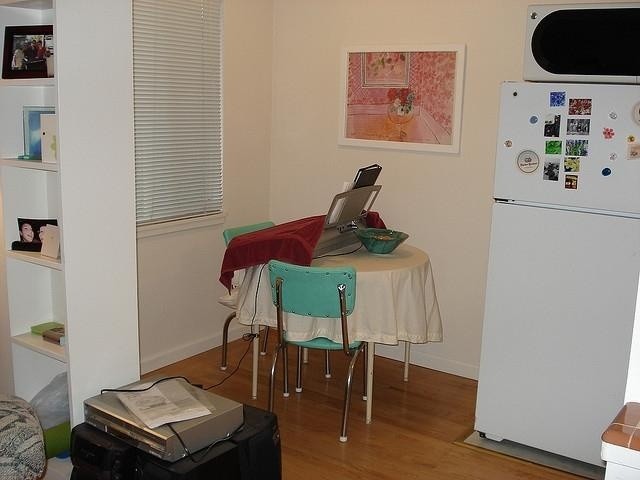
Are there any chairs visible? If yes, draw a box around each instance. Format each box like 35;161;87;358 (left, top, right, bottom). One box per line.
221;220;276;369
266;257;368;443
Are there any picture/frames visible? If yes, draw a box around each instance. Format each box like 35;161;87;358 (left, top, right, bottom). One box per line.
338;41;468;157
1;23;53;79
17;216;57;245
37;113;56;164
23;105;55;161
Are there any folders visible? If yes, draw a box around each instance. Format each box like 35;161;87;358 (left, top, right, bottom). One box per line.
354;164;383;189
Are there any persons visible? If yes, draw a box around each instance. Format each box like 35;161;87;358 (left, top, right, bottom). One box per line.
13;44;24;70
35;41;46;60
39;226;46;243
19;222;34;243
27;40;36;59
23;43;27;54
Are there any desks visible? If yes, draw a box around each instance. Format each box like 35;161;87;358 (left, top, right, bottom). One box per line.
216;242;443;423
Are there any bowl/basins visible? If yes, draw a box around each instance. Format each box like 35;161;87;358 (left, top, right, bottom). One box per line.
354;225;410;255
